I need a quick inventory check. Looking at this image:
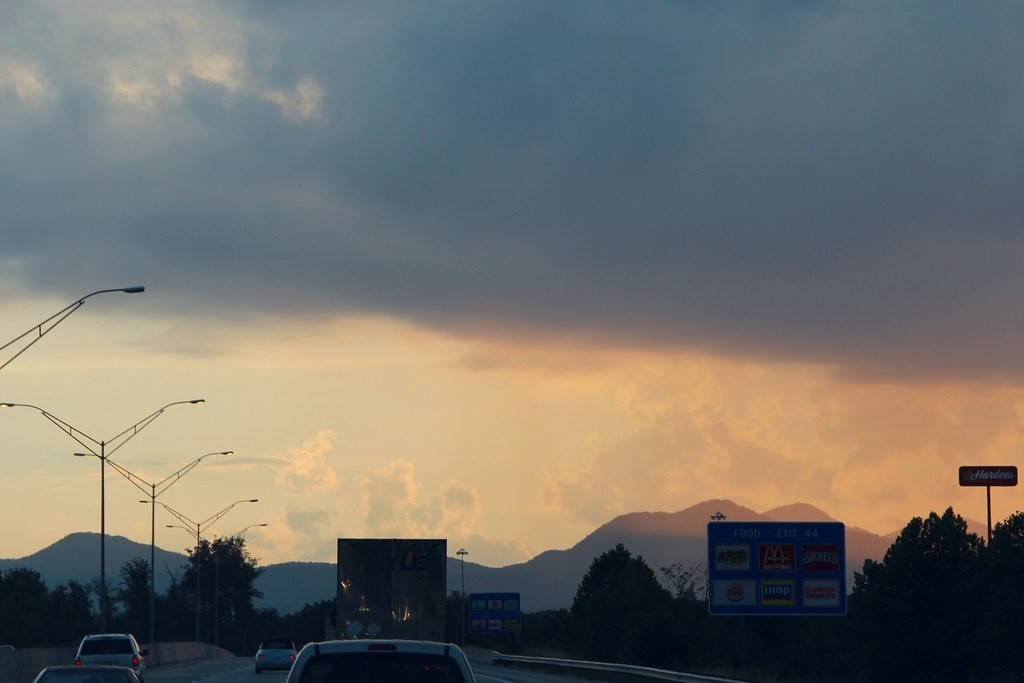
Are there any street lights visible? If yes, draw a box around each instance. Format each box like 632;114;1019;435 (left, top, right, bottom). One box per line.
455;547;469;649
1;397;207;636
137;496;259;642
72;451;235;642
165;523;268;646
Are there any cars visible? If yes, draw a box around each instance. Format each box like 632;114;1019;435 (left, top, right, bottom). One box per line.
286;639;476;683
32;665;141;683
0;644;15;650
255;638;298;674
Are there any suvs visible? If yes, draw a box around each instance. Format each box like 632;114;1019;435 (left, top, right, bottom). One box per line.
73;633;150;683
